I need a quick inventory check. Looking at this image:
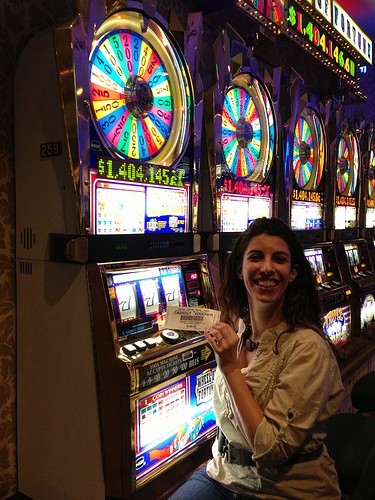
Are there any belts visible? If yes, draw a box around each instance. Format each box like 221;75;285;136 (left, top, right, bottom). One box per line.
217;425;324;467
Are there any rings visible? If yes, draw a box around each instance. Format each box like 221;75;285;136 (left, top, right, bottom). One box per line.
217;337;225;343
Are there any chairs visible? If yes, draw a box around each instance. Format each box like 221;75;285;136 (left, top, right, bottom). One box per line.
325;369;375;500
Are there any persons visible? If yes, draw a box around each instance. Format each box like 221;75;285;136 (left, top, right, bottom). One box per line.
167;216;345;500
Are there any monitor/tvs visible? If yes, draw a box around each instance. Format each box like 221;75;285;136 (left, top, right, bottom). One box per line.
104;264;208;342
343;242;366;274
303;247;330;285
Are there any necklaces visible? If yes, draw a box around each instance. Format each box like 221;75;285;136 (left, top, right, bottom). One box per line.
243;326;260;352
